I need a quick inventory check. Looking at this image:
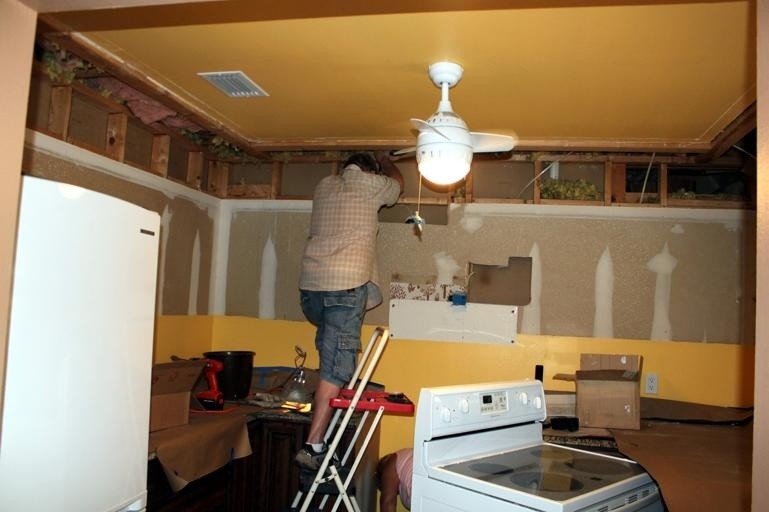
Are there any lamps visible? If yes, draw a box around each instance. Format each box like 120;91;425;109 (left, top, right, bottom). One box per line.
269;345;312;410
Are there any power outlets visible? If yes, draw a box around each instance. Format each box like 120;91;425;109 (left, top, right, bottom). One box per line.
644;374;658;394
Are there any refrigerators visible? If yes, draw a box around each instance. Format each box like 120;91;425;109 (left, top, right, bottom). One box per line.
1;174;162;512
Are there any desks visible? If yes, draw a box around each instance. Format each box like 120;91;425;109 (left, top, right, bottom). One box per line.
146;382;381;511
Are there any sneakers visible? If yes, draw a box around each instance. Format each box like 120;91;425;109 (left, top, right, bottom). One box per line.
296;443;342;472
298;473;355;495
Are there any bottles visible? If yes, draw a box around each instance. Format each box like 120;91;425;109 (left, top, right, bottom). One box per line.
281;368;309;404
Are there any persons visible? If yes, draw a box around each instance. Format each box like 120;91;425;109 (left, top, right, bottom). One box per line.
296;142;405;474
367;449;416;511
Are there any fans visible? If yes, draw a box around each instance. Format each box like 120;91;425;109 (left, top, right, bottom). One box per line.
390;60;519;235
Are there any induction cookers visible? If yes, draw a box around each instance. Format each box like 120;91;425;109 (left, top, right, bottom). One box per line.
440;443;651;505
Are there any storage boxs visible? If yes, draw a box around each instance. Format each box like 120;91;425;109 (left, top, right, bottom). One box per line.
551;349;647;435
148;357;209;435
